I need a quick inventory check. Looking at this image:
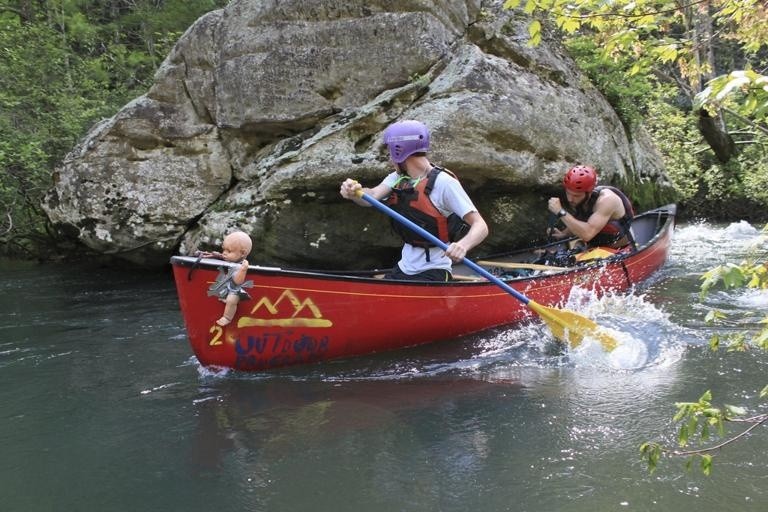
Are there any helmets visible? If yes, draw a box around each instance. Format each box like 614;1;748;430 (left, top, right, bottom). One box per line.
381;120;431;164
564;164;598;193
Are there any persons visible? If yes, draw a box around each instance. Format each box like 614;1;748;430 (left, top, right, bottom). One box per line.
213;231;253;326
545;164;636;267
338;119;490;283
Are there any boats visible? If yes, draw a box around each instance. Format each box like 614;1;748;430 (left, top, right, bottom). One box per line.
168;202;676;373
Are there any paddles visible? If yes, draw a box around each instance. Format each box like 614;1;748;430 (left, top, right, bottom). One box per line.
350;182;617;357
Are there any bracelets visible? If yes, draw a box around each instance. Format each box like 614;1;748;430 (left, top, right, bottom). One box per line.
557;208;567;217
